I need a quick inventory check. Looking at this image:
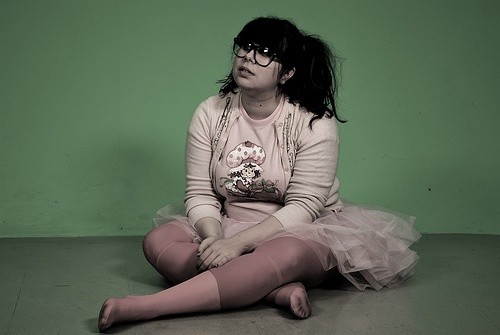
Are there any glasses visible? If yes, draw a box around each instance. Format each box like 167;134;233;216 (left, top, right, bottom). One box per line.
232;37;282;67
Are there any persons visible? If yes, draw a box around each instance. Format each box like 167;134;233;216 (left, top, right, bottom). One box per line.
96;15;360;327
